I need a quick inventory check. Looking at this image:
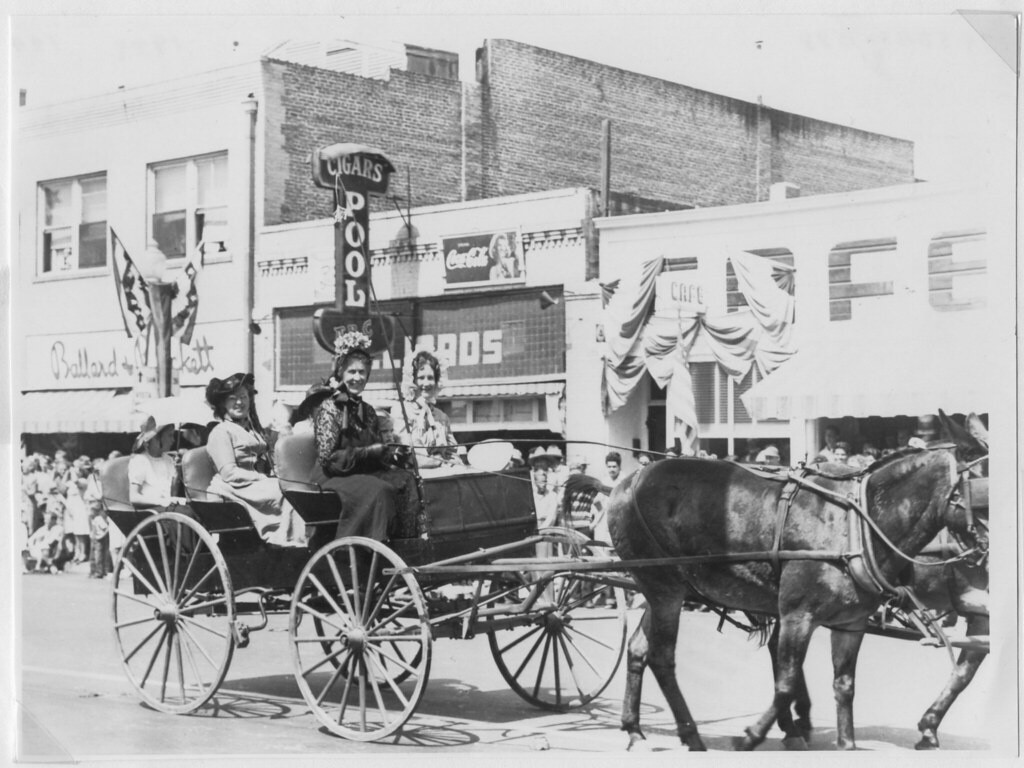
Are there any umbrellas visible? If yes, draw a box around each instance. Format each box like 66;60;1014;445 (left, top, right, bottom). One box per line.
135;396;223;453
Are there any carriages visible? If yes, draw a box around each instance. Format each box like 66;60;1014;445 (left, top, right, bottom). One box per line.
102;436;988;755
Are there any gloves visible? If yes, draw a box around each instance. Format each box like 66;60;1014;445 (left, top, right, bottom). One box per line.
394;446;412;461
367;443;390;457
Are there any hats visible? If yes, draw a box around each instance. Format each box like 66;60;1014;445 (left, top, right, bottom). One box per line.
136;415;172;449
181;423;220;429
401;344;449;400
205;373;254;400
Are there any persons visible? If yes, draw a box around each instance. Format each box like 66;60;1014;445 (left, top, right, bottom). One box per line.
593;419;910;526
291;381;336;433
205;369;317;549
374;410;416;474
558;454;612;558
313;350;427;603
128;416;206;555
528;446;558;492
488;232;518;279
532;469;560;558
546;444;569;493
19;449;123;578
389;351;465;468
179;423;205;449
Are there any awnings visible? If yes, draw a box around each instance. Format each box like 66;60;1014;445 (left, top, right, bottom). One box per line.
20;391;154;432
739;315;990;420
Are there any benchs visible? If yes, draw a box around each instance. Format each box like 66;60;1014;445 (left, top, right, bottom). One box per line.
182;446;257;536
102;450;180;555
270;432;409;542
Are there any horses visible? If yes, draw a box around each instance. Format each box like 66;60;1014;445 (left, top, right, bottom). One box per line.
561;408;990;752
